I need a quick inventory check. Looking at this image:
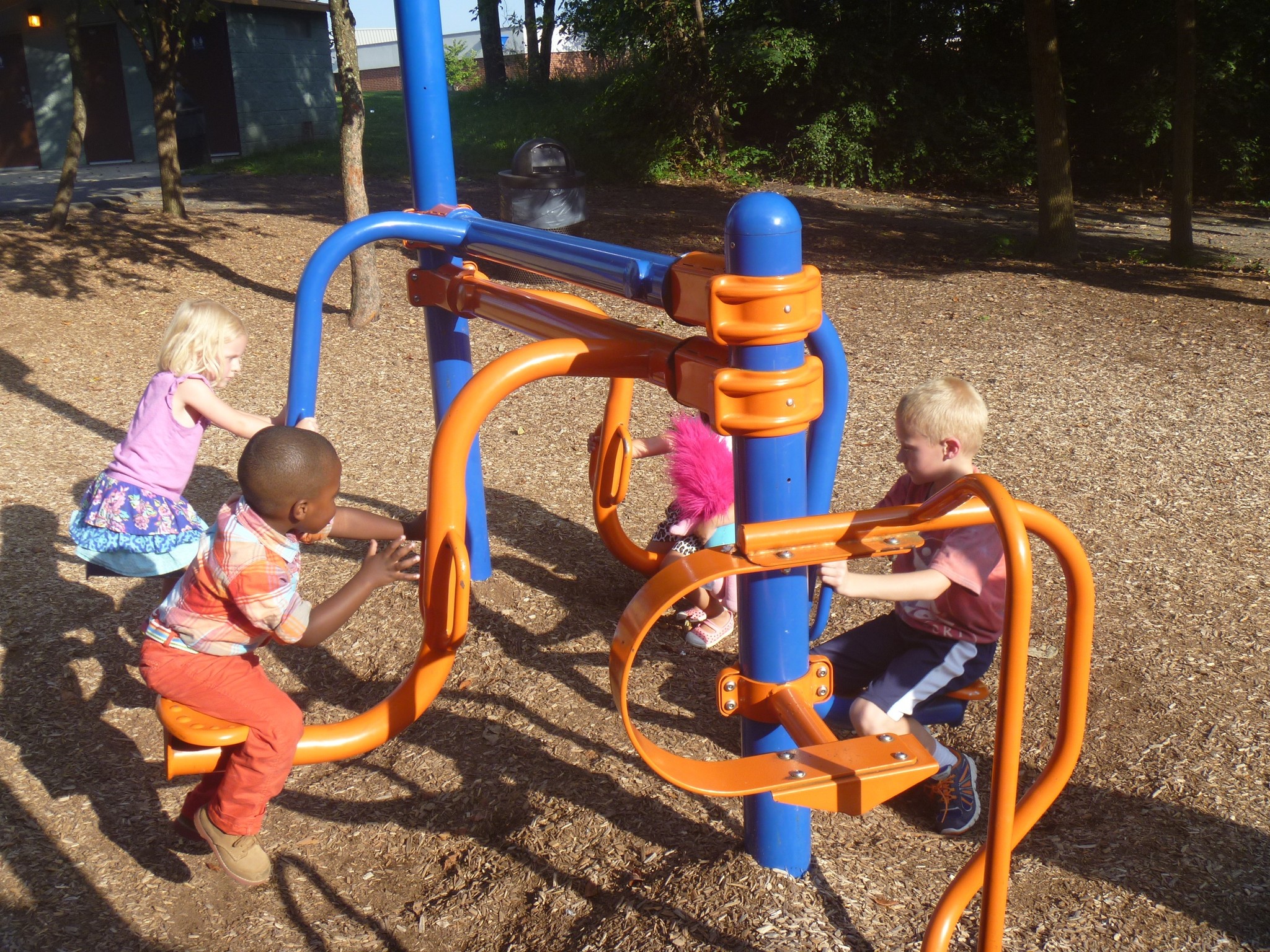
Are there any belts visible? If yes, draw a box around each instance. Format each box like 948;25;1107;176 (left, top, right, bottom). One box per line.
145;608;201;654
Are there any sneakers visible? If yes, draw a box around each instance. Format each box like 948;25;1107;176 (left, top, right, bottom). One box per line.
193;801;272;886
172;813;207;842
922;740;980;836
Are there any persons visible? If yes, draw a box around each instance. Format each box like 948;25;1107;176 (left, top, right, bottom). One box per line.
138;426;420;885
68;298;291;581
584;394;741;651
803;375;1015;834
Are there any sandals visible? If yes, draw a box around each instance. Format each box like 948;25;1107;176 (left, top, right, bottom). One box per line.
676;606;707;624
684;611;735;648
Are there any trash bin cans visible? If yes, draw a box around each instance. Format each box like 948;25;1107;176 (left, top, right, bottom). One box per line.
499;137;587;286
